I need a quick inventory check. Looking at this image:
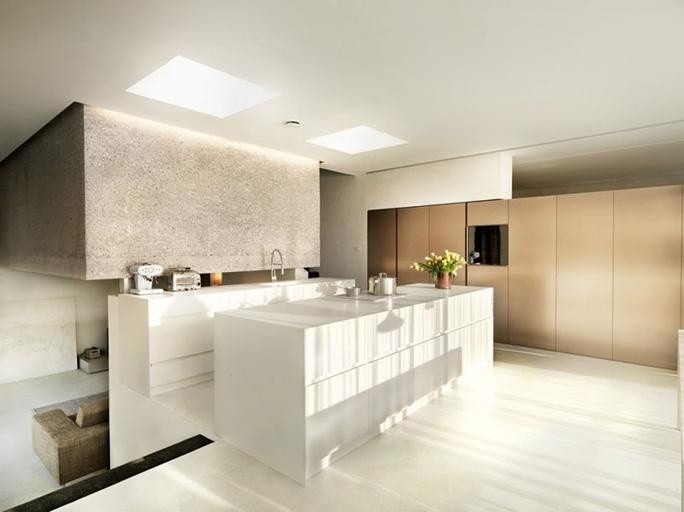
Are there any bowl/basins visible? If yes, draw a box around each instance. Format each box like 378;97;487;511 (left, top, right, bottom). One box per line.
342;286;362;298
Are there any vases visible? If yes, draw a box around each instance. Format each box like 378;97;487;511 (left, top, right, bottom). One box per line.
436;270;451;290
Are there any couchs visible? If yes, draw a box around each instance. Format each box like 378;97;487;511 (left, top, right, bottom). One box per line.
32;398;109;485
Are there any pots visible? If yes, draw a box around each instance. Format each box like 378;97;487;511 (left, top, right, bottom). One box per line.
368;273;396;296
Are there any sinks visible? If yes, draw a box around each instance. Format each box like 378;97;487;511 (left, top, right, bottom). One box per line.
261;280;314;287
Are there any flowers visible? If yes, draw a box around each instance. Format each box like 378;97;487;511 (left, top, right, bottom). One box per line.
409;249;468;274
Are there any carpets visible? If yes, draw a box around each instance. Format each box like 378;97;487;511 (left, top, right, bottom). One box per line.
33;390;108;417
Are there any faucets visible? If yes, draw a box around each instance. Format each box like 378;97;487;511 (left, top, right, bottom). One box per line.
271;249;284;281
